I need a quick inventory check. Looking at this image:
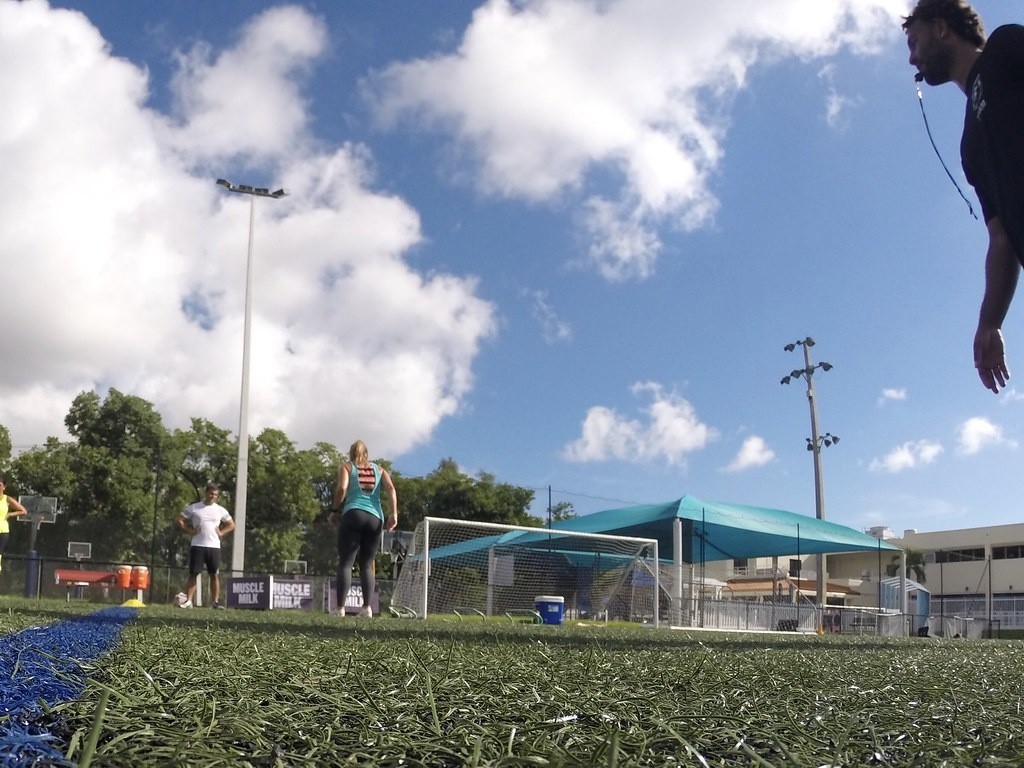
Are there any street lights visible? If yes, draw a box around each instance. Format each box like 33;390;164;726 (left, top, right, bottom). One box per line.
779;336;840;606
216;176;292;582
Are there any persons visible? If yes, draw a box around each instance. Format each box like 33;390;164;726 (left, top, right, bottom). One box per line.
0;476;27;575
903;0;1024;395
177;486;235;610
329;440;397;617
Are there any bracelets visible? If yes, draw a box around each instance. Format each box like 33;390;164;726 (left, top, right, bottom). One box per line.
331;509;338;513
389;514;398;517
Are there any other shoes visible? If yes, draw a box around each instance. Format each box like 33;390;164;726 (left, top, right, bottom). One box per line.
328;607;345;618
357;606;372;618
212;602;224;610
178;600;193;609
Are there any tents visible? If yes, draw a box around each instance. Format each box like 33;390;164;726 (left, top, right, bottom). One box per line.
398;532;686;618
493;496;908;636
599;570;665;586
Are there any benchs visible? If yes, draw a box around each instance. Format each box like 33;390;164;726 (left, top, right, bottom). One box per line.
849;617;879;631
54;569;150;603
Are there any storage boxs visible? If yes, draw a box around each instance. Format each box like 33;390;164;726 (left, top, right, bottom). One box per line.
534;596;564;625
